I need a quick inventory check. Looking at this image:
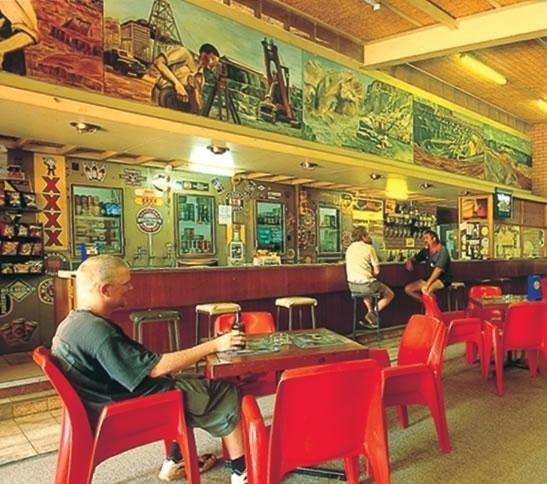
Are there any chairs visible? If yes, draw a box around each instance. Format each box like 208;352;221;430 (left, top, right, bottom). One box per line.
489;299;547;393
379;312;452;457
213;309;297;460
31;344;202;484
421;291;493;384
465;283;508;363
231;358;398;483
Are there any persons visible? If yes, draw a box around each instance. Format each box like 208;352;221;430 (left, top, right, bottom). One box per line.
150;42;220;113
52;253;249;483
344;226;395;329
0;0;41;77
403;229;454;305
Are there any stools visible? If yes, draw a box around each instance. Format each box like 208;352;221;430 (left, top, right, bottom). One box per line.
191;301;241;347
128;308;182;357
275;296;318;332
349;291;382;343
446;280;466;315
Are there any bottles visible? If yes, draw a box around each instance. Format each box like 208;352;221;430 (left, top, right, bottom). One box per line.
399;248;403;260
227;223;244;260
232;310;245;349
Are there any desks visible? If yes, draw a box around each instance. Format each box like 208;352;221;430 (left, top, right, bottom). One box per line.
470;293;528;324
198;326;369;463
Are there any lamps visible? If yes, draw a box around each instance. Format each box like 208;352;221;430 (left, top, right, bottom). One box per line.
418;183;432;190
301;160;323;172
208;146;229;157
370;173;383;180
72;122;103;137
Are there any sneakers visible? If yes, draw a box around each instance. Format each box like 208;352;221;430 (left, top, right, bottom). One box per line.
158;453;216;481
360;317;372;329
365;311;378;328
231;469;248;483
